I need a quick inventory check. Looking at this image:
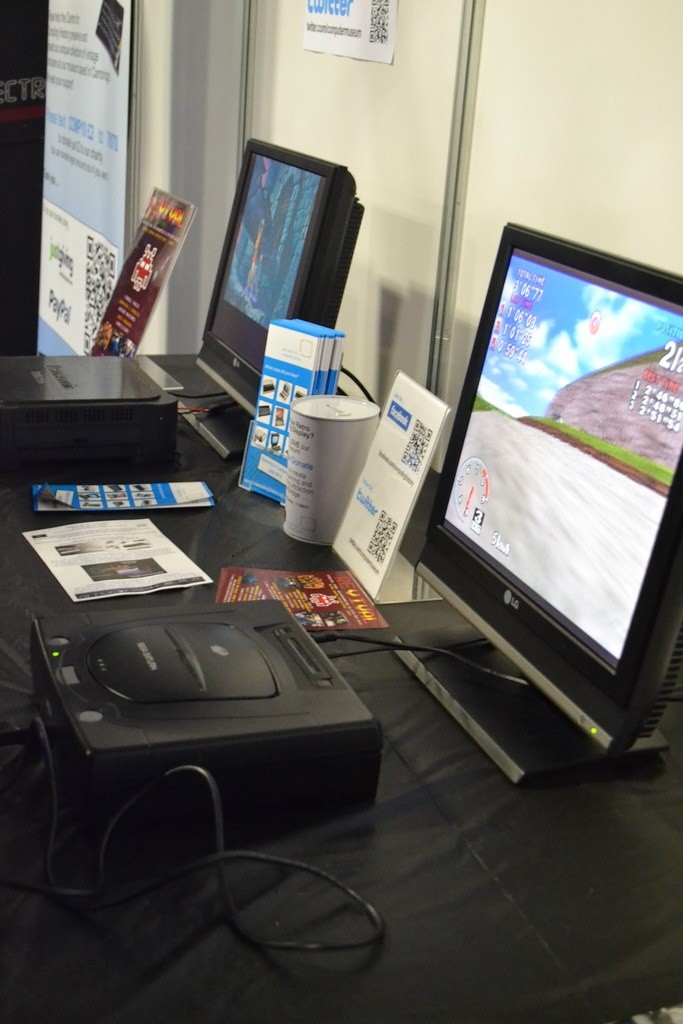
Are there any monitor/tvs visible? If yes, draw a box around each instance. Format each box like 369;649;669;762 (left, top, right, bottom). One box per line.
392;221;683;786
177;138;365;458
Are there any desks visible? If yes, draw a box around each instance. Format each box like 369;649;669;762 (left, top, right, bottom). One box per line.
1;355;683;1024
